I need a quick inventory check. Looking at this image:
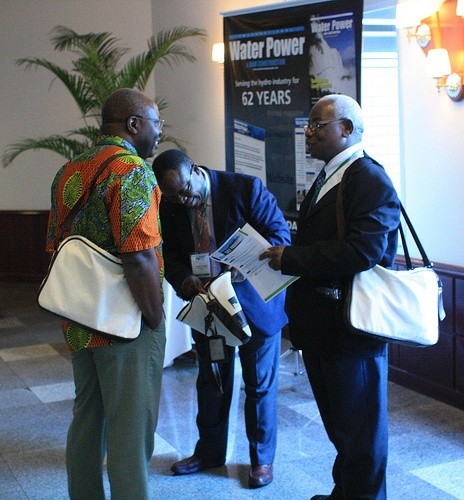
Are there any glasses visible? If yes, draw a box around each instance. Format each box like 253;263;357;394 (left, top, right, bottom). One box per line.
304;117;349;132
164;165;192;204
125;114;165;130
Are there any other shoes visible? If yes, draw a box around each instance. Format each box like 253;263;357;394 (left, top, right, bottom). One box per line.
311;494;332;500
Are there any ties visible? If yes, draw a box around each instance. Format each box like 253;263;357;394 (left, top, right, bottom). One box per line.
312;170;327;208
195;201;210;253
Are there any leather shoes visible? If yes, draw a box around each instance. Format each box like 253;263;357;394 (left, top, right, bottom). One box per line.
249;463;272;487
171;453;225;475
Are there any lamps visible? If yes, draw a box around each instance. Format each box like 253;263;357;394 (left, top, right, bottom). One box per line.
396;0;464;102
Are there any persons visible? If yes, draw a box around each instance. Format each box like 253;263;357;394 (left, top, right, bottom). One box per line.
152;148;291;487
43;89;166;500
258;95;401;500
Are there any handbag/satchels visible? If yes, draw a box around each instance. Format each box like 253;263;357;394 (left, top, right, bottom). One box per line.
342;261;446;348
36;236;143;340
176;271;251;347
